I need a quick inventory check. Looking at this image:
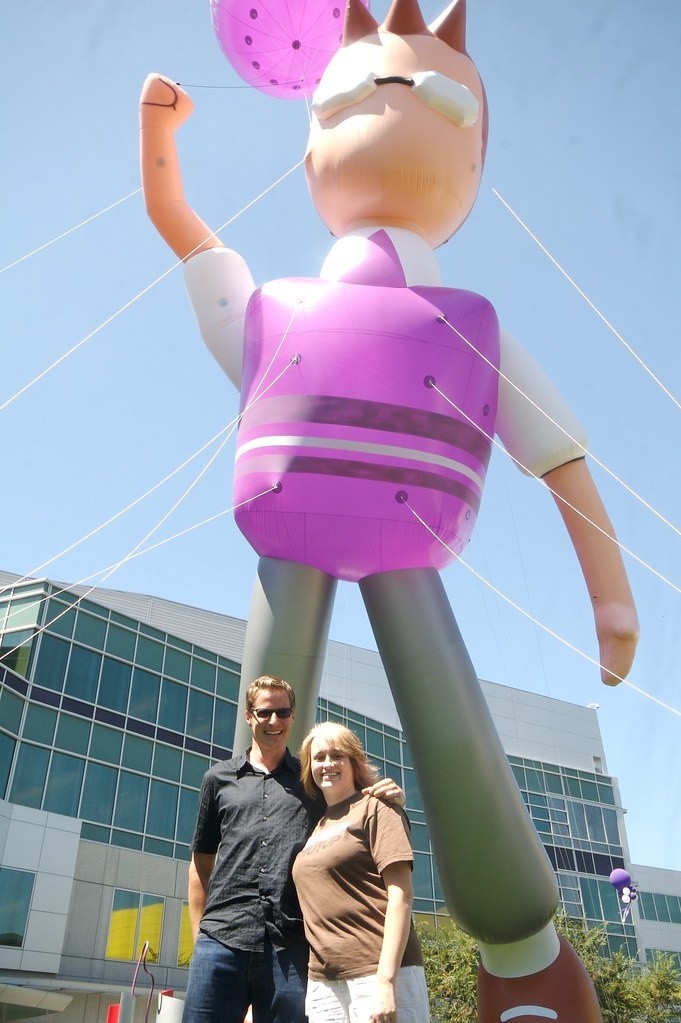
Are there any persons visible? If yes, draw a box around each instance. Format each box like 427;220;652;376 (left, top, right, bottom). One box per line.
182;673;407;1023
292;720;431;1023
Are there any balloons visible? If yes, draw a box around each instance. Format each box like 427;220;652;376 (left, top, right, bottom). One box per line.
610;869;637;903
209;0;371;100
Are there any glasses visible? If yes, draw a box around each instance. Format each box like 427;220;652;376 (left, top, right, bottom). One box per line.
250;706;292;718
